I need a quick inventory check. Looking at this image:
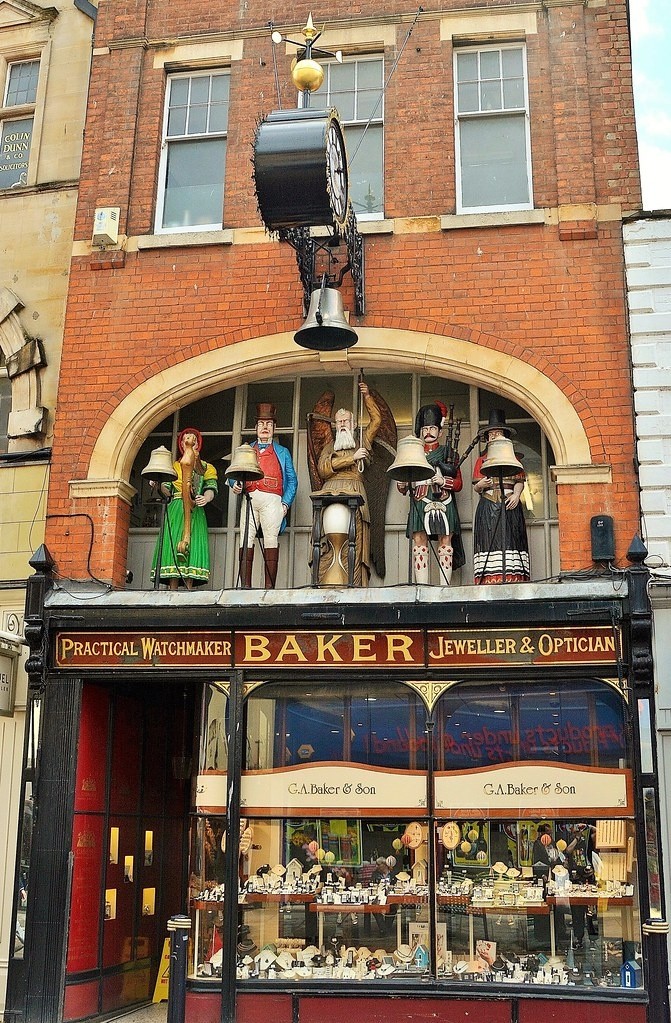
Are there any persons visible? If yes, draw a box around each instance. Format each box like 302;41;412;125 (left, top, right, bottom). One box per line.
565;823;600;951
533;824;570;943
478;942;493;965
396;405;466;586
472;409;530;585
150;428;218;590
368;857;399;936
317;383;382;590
228;404;298;589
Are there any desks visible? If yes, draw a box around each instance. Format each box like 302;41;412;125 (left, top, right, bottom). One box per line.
193;882;633;968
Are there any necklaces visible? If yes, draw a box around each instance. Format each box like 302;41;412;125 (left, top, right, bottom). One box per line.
381;966;391;972
456;963;468;971
491;963;505;969
237;941;256;951
257;952;273;963
397;949;413;958
280;956;290;967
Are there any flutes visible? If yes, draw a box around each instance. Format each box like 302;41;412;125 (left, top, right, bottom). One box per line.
430;401;482;499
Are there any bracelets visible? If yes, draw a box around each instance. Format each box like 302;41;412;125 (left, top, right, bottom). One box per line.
576;837;579;841
